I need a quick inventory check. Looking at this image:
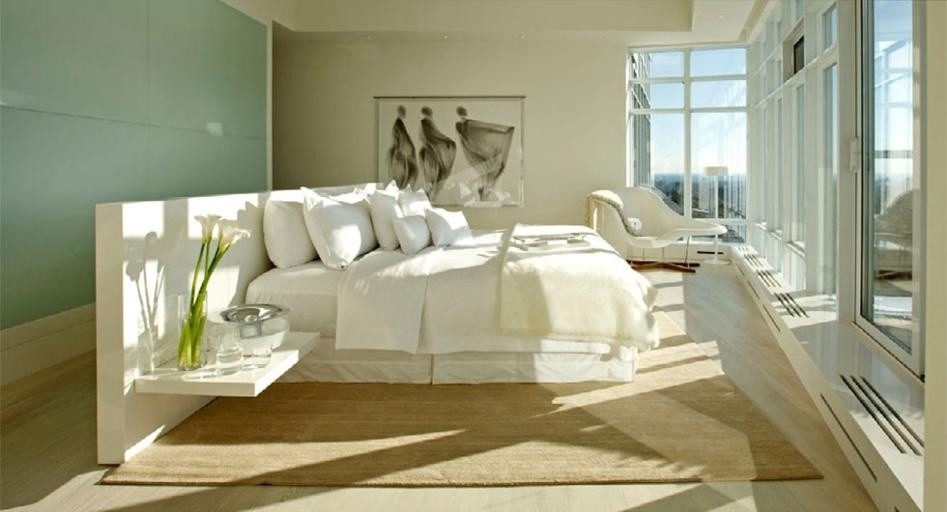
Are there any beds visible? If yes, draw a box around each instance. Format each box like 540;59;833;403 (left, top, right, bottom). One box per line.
243;222;661;386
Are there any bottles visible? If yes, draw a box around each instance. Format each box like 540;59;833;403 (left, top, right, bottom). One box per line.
214;323;243;376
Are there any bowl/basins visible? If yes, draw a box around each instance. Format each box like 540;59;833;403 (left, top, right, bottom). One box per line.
204;314;290;356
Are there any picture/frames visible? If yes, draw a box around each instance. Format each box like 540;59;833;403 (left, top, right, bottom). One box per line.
372;94;525;209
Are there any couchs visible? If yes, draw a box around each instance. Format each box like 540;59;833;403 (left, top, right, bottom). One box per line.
583;185;729;273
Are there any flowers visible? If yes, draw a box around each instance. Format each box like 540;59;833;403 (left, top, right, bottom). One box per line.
176;213;252;367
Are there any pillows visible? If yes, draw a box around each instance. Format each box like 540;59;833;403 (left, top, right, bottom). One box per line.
300;185;380;273
423;207;475;248
390;187;433;256
261;195;317;271
369;179;402;251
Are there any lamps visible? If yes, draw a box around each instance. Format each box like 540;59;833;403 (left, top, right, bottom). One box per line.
703;165;731;266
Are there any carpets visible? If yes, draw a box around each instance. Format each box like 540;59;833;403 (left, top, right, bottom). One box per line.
92;302;827;488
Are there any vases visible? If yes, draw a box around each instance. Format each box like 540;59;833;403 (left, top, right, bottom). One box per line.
175;293;210;374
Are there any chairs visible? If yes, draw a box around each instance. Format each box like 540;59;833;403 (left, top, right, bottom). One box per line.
872;189;912;280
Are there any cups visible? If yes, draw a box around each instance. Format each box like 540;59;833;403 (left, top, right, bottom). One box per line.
248;339;273;369
